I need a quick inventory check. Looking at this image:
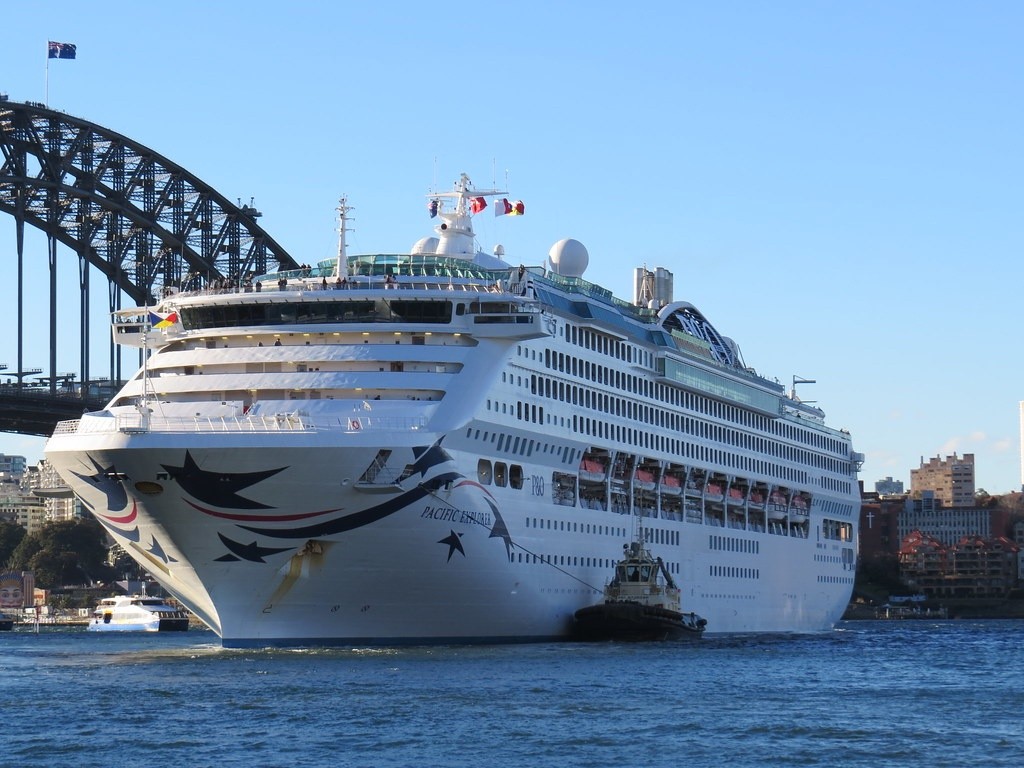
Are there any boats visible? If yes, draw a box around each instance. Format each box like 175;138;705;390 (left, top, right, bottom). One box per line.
623;467;656;490
660;476;683;497
579;457;608;483
568;481;712;641
769;490;788;520
83;594;189;632
791;494;809;523
749;489;764;510
703;482;723;503
725;486;745;507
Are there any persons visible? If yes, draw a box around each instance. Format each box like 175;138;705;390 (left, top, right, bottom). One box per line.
301;262;312;277
278;277;287;291
116;315;142;332
384;276;399;290
244;280;262;292
209;277;234;294
322;276;359;290
688;612;698;628
518;264;524;282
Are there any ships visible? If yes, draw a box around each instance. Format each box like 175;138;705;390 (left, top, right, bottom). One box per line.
38;170;867;648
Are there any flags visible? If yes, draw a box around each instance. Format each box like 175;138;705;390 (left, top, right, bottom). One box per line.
148;309;179;328
494;199;524;216
428;201;438;219
46;39;76;59
470;196;488;214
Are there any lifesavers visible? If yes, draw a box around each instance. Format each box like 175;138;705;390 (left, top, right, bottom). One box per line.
352;420;359;429
